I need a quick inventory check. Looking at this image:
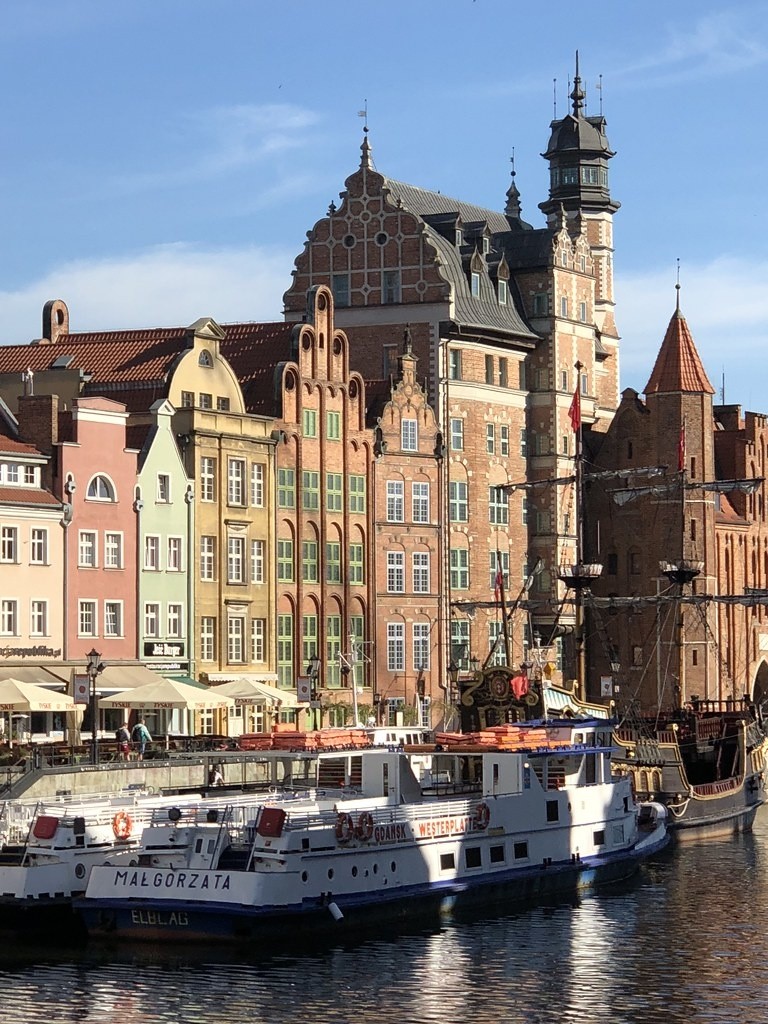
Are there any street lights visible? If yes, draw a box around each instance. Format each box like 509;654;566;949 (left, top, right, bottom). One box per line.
85;647;108;765
306;652;322;731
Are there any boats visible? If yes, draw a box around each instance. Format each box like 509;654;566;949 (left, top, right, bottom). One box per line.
445;362;768;843
0;742;675;947
403;713;622;754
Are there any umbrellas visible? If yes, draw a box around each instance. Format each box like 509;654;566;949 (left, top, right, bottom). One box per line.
66;668;85;746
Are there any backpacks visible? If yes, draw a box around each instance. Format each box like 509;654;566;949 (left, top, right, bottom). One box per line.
116;728;128;742
132;727;141;742
208;771;215;782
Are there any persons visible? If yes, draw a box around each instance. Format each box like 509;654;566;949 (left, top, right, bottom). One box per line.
208;766;224;787
118;722;131;761
131;719;153;762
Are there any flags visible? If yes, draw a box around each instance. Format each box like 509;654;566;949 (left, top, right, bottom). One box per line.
567;389;580;432
494;562;501;601
678;426;684;470
510;671;528;701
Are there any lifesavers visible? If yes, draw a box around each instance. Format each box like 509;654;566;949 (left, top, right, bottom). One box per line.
113;812;132;839
355;812;373;840
476;804;490;830
335;813;355;844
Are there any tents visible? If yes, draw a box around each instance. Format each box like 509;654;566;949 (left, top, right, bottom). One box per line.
204;679;309;733
0;678;74;748
97;678;235;750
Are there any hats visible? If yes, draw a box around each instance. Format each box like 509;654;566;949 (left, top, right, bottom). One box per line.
212;764;219;767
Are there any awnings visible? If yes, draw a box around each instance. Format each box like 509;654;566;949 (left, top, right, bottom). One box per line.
203;671;278;681
0;666;67;691
45;665;164;696
